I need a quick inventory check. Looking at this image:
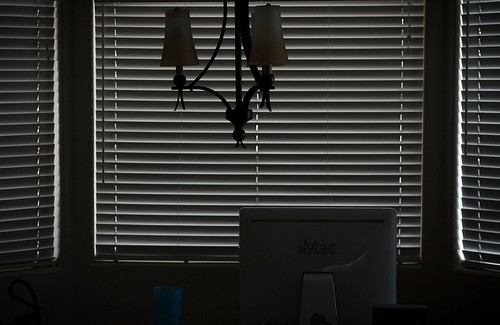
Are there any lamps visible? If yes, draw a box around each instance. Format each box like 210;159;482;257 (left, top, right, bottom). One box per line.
161;1;289;149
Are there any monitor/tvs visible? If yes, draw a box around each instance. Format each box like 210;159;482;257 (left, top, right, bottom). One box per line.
237;204;400;309
